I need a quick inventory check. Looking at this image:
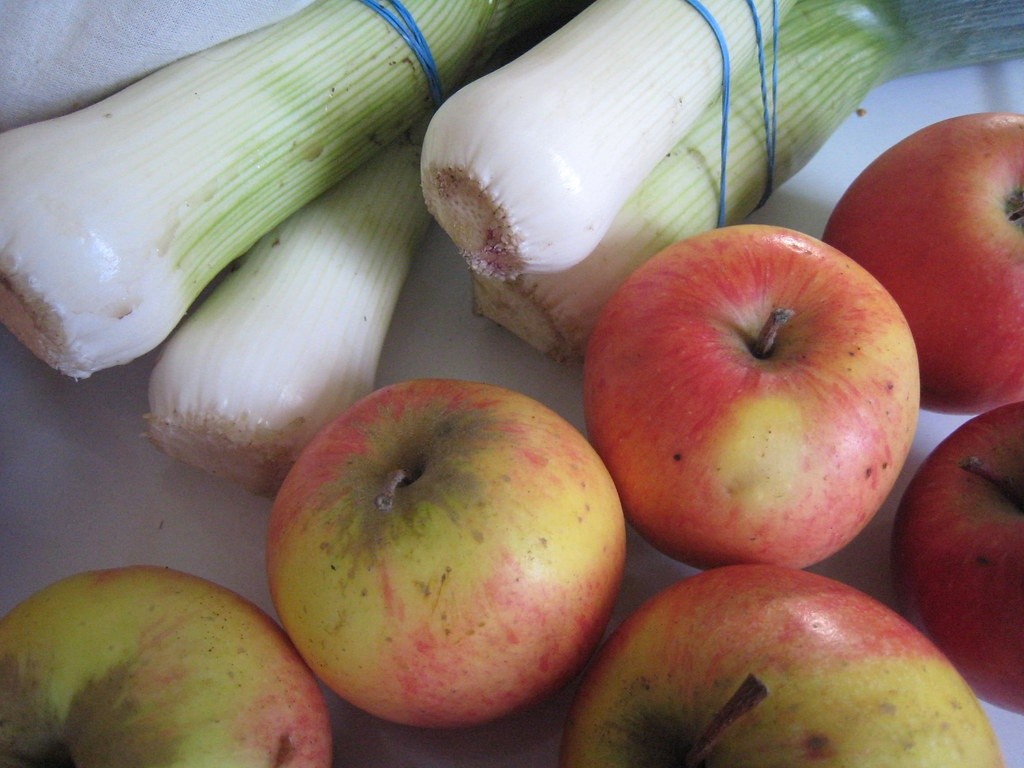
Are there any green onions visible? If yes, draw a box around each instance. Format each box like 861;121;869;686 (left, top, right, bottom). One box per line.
0;0;1024;497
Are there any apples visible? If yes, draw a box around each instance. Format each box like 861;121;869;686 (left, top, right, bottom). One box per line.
0;112;1024;768
